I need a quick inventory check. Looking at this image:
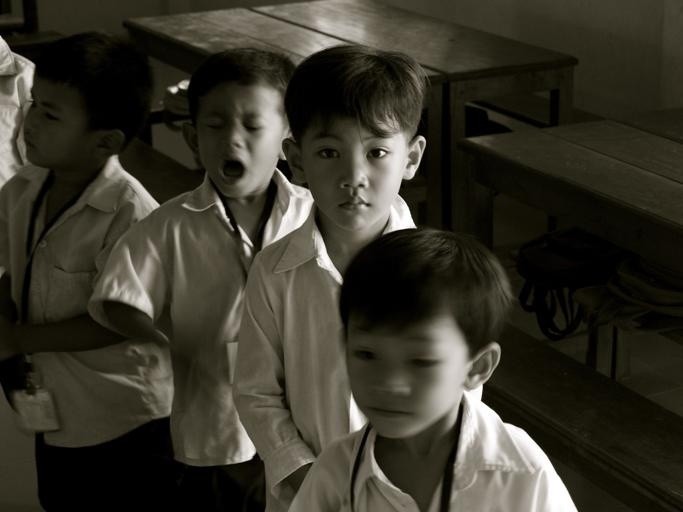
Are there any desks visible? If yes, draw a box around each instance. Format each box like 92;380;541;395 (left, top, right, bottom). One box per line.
252;2;579;248
458;114;681;358
122;1;445;236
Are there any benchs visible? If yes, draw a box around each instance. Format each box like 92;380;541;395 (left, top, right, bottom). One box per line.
478;321;681;509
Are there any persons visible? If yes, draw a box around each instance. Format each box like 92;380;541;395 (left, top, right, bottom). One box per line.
288;228;581;512
1;0;50;201
0;29;181;510
230;45;430;512
88;47;313;511
